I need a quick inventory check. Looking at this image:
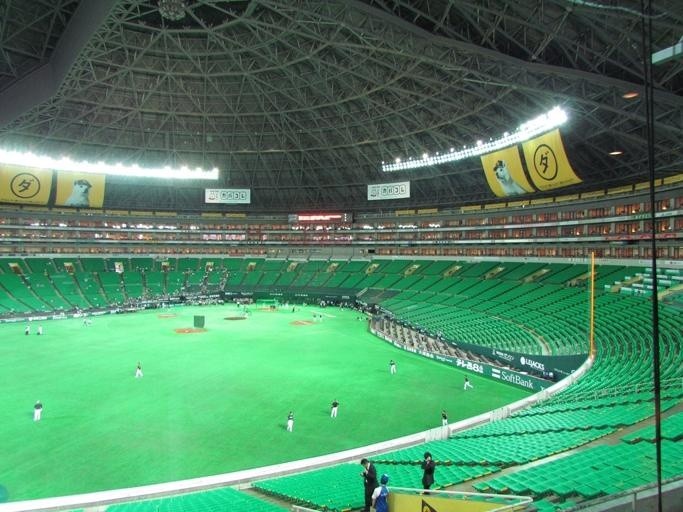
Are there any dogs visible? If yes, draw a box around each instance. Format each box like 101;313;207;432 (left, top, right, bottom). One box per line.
65;179;92;207
493;160;528;196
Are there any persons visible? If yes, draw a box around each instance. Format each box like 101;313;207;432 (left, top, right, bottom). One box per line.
464;374;473;390
360;459;377;512
372;474;389;512
422;452;434;489
442;411;448;426
287;411;294;432
331;398;339;417
135;363;142;376
33;402;42;421
389;360;396;374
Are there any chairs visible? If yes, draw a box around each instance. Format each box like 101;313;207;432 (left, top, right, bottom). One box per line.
55;458;363;509
368;256;681;396
362;396;681;510
0;254;368;315
